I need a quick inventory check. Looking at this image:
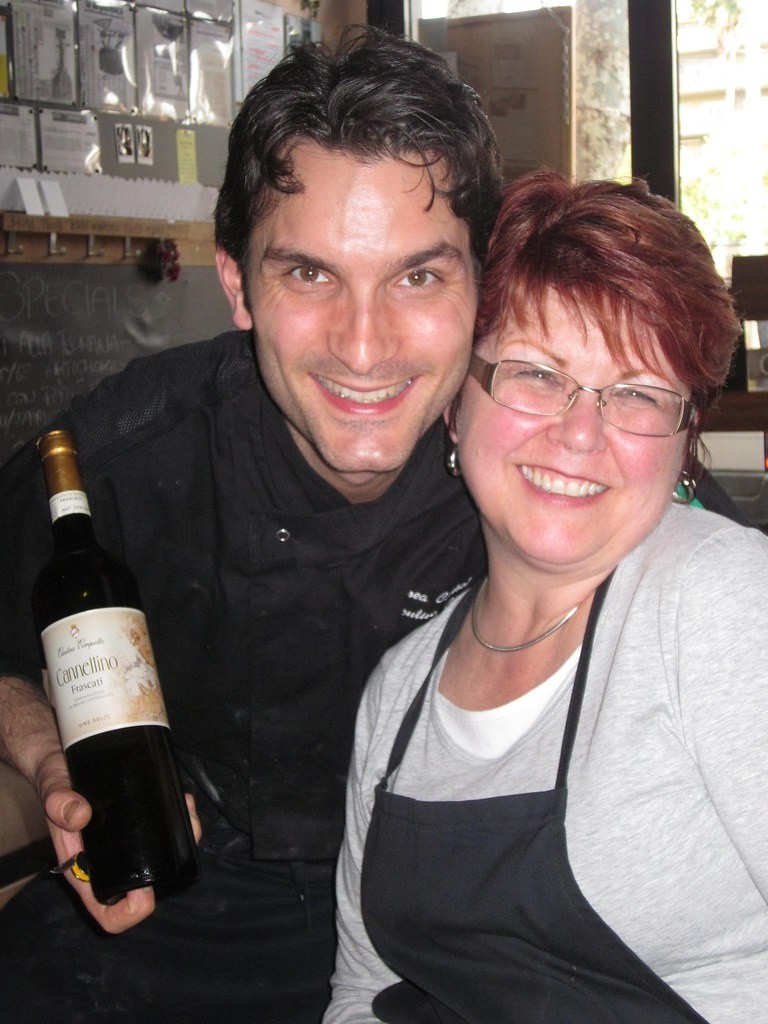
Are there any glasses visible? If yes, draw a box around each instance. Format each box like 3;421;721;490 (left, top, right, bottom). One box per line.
469;349;697;437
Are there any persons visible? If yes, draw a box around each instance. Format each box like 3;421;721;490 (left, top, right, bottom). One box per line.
0;21;756;1024
322;173;768;1023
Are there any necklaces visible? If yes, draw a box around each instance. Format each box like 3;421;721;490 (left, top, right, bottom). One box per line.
472;577;579;651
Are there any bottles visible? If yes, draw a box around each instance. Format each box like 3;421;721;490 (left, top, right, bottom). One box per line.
32;428;201;905
